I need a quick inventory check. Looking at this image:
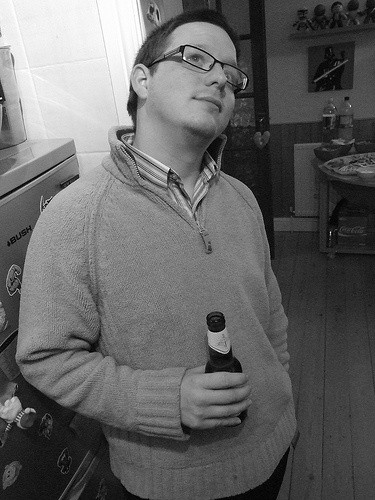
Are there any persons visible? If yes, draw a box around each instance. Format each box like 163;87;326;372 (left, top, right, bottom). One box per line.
15;7;301;500
311;45;346;91
292;0;375;31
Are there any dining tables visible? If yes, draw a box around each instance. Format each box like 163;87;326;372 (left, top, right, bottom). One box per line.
319;151;375;260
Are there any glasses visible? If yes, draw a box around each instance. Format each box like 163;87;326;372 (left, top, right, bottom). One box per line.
147;44;249;94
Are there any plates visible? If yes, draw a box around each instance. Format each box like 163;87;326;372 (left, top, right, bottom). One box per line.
325;153;375;176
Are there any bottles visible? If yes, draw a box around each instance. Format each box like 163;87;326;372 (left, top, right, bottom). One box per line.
339;96;354;138
321;97;337;139
205;311;247;428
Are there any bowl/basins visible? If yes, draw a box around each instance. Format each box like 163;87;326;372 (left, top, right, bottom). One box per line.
355;166;375;179
314;144;350;161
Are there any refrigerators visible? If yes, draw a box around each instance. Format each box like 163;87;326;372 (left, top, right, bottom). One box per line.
0;138;126;500
